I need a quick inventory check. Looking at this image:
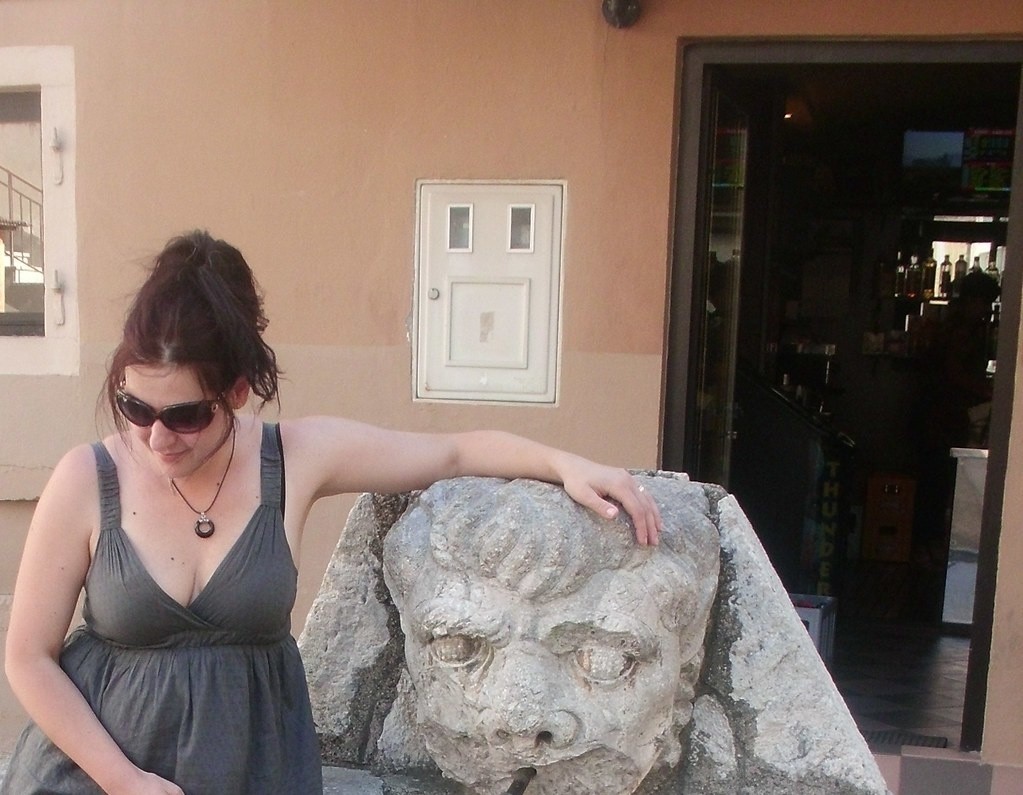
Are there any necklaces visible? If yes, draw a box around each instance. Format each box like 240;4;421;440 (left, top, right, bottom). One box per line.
166;426;237;536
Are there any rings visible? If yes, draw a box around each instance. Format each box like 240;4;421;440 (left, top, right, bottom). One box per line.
638;485;647;494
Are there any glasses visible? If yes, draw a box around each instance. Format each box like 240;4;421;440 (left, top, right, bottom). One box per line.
114;382;230;435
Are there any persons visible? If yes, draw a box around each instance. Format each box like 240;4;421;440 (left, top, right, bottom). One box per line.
915;271;1001;571
4;230;664;795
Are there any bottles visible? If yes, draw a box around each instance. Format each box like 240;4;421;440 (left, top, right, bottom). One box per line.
953;254;967;299
969;257;982;274
938;254;952;300
905;248;922;300
984;256;998;283
892;248;905;298
921;248;937;299
